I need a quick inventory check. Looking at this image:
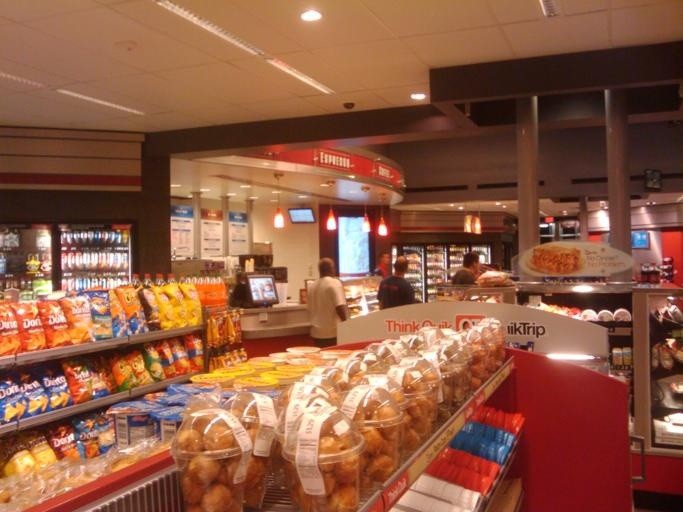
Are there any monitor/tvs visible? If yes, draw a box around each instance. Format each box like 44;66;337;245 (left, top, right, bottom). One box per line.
247;274;280;305
288;208;316;224
631;231;649;248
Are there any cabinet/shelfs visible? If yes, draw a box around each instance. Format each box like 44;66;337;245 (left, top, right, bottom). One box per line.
0;294;248;510
515;287;682;497
391;233;506;304
0;220;133;285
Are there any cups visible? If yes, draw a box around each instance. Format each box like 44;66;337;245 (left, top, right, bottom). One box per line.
167;320;504;511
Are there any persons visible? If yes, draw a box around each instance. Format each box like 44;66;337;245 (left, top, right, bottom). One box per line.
371;249;391;278
229;273;246;308
377;255;415;309
307;257;348;349
451;251;480;284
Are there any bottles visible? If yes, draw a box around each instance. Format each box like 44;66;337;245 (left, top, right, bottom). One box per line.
131;273;227;309
58;228;128;289
640;261;659;281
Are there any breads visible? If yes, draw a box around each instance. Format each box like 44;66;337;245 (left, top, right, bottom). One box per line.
275;317;507;496
531;243;586;274
283;405;364;511
221;391;282;511
170;408;254;512
